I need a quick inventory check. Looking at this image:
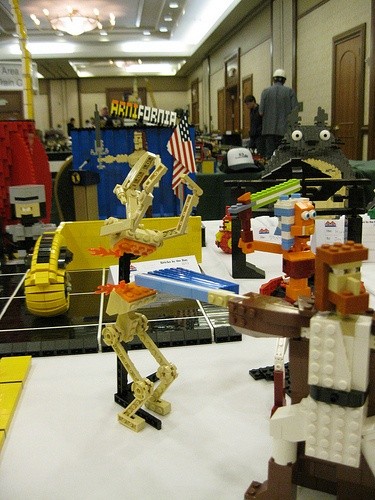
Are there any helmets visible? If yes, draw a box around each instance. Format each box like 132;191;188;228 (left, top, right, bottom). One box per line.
272;69;286;80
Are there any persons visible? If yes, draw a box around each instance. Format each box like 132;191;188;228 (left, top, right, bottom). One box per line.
56;105;125;129
244;95;266;158
258;69;298;160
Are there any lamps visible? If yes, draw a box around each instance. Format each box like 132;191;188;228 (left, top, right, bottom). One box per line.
50;18;101;37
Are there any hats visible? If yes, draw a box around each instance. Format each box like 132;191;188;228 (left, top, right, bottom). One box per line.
227;148;258;171
